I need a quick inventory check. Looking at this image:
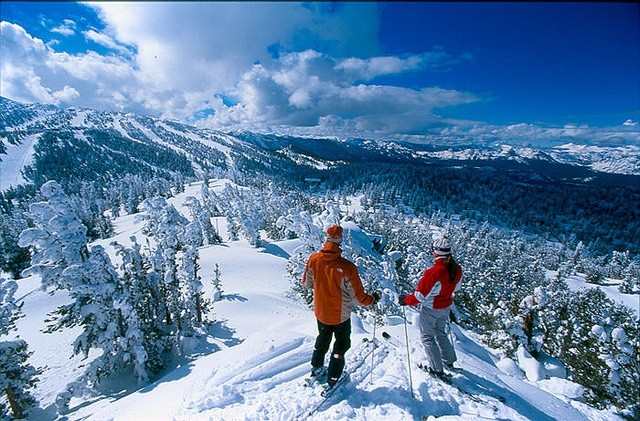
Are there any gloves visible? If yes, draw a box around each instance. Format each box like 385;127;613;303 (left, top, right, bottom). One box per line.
399;295;407;306
372;290;382;304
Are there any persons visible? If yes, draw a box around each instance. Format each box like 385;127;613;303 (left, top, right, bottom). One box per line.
398;237;463;376
302;225;382;388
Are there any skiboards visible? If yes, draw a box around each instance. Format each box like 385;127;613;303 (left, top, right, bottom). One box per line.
302;338;377;417
382;331;505;411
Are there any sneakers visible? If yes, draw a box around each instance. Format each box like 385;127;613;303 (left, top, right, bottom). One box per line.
442;359;453;371
328;368;347;384
312;366;328;377
422;364;443;374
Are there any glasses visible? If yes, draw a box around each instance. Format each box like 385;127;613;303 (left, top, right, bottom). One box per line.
431;243;451;252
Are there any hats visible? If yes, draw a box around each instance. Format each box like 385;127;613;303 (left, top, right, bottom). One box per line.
326;226;343;243
432;239;451;255
